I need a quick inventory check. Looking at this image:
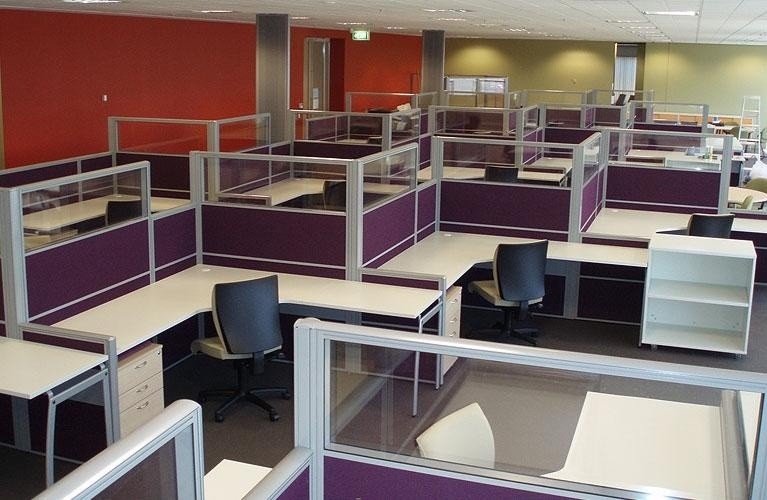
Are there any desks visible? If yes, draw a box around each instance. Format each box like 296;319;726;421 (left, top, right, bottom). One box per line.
1;89;766;500
